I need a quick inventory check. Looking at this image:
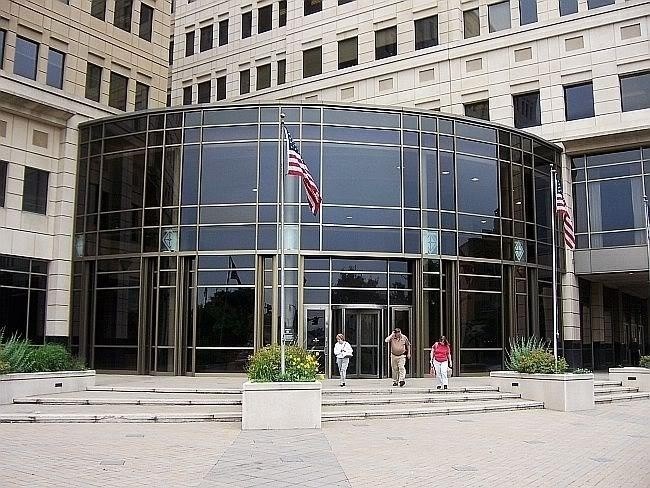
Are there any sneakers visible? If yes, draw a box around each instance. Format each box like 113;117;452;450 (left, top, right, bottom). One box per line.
393;381;405;387
340;382;346;388
437;385;448;389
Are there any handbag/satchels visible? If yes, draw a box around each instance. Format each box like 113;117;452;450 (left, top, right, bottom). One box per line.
342;351;353;358
429;362;436;377
447;365;452;377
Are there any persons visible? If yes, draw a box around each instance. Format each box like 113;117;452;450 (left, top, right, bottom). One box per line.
430;335;452;390
384;328;412;388
333;333;353;386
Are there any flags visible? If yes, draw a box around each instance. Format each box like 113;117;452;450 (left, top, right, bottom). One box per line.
554;174;576;250
228;256;242;286
282;122;322;217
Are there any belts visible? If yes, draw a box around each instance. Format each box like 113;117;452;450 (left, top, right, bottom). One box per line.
392;353;403;356
